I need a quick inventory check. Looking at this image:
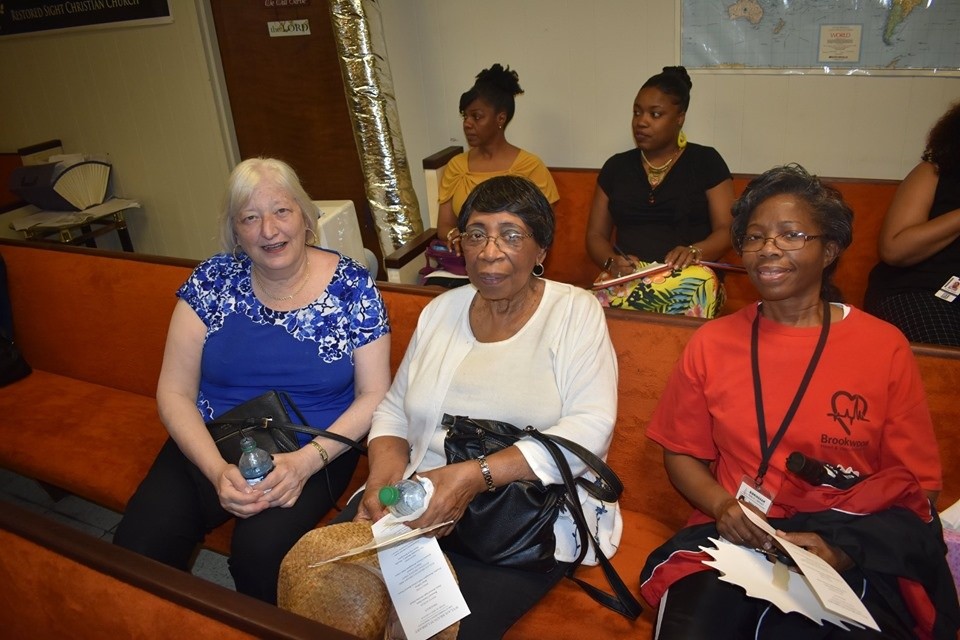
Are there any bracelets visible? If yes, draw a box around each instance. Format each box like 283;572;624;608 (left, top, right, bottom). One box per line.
690;245;697;263
480;455;496;492
446;227;458;240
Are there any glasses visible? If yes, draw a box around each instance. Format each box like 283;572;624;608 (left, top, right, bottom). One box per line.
737;231;824;253
466;228;535;249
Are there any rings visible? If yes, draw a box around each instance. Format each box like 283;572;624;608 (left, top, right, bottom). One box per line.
617;272;621;278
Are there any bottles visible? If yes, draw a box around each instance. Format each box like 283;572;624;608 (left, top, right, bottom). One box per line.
239;436;274;495
378;479;427;518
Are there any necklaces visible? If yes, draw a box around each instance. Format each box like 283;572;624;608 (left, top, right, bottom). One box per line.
638;144;681;204
252;250;310;302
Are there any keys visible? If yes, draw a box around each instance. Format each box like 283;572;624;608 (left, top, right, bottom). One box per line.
755;547;794;567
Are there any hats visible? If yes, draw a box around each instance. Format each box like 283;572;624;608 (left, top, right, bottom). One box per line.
277;518;460;640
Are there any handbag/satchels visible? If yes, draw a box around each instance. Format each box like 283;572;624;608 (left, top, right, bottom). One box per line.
420;238;467;276
436;412;643;624
201;388;366;506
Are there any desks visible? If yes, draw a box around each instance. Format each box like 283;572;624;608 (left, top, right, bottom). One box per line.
29;211;135;251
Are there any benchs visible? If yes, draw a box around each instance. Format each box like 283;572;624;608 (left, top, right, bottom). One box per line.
0;147;960;640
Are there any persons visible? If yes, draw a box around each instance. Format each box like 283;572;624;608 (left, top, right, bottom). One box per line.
865;104;959;350
313;174;620;640
646;163;942;640
112;159;392;606
586;66;736;320
421;63;560;289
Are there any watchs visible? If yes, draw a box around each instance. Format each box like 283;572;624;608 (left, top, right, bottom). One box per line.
309;441;329;468
603;257;615;274
